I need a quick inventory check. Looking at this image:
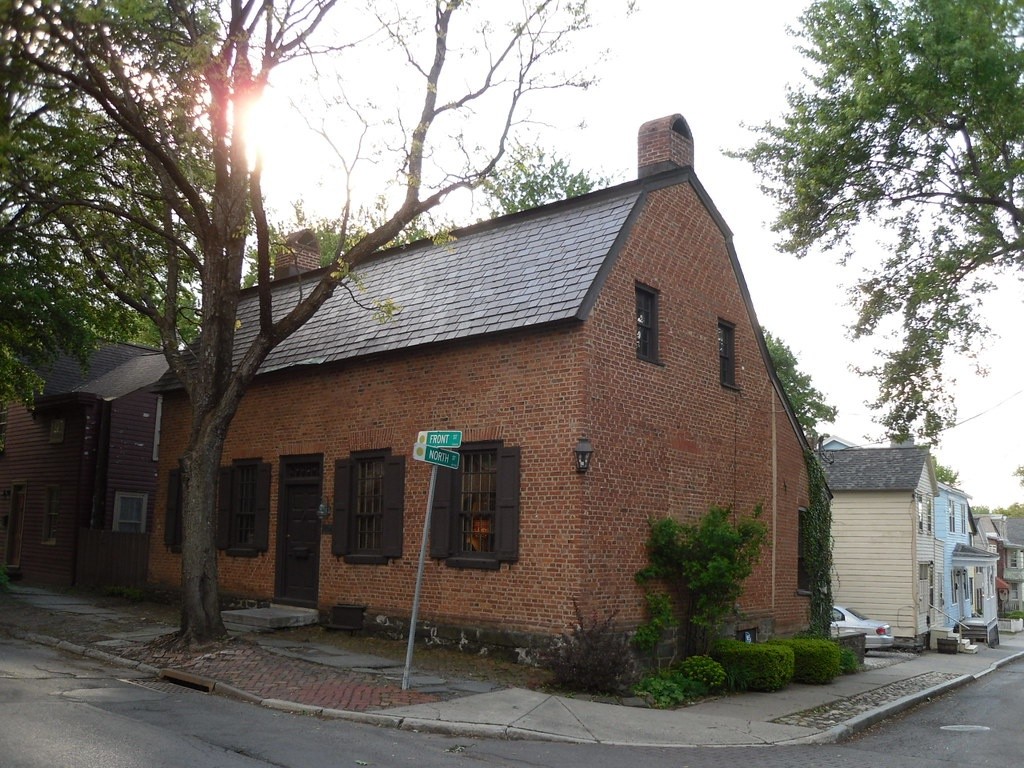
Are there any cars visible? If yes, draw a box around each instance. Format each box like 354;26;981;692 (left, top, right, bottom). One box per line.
829;606;894;653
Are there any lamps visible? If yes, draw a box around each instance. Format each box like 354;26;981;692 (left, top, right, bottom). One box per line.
963;570;967;574
572;436;592;474
315;495;332;535
929;560;934;569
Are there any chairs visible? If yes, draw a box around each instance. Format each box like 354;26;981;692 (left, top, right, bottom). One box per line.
323;605;366;637
